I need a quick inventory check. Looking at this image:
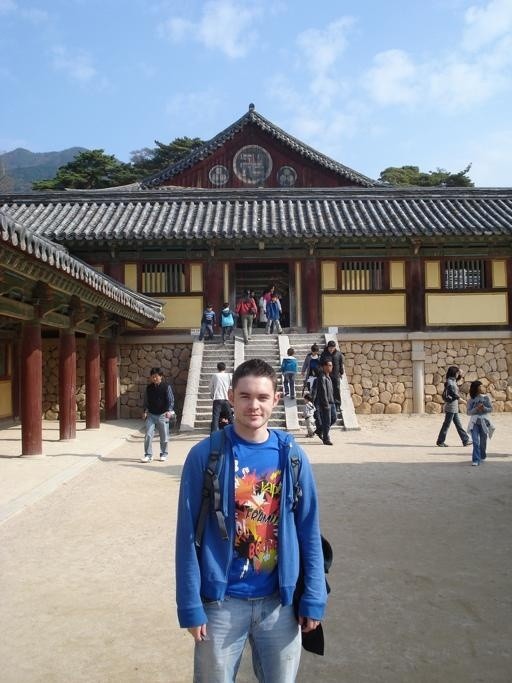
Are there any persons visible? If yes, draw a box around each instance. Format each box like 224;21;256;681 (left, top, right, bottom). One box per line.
173;356;330;682
198;284;283;345
463;379;495;467
209;359;235;432
435;365;473;448
280;348;298;401
302;340;346;446
142;368;177;461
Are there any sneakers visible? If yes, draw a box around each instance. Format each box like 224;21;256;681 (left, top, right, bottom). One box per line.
158;455;167;460
141;457;152;462
